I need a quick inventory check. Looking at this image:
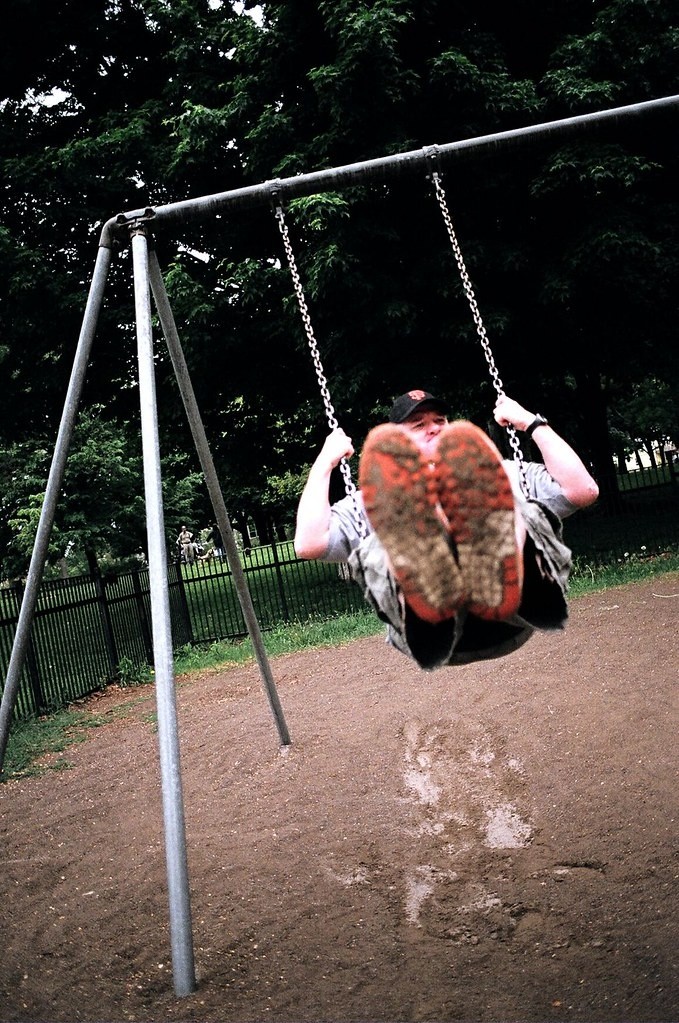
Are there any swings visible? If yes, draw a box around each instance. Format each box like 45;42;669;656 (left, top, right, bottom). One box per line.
273;173;539;667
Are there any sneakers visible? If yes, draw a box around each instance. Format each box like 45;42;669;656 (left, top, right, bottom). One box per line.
361;423;465;620
430;419;524;620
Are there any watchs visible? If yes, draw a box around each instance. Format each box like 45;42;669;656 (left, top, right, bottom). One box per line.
524;413;548;440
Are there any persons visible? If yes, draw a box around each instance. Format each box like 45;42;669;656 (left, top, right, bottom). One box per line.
294;395;598;671
179;525;195;566
206;525;225;564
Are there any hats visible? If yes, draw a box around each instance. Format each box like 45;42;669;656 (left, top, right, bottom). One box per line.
390;389;441;423
